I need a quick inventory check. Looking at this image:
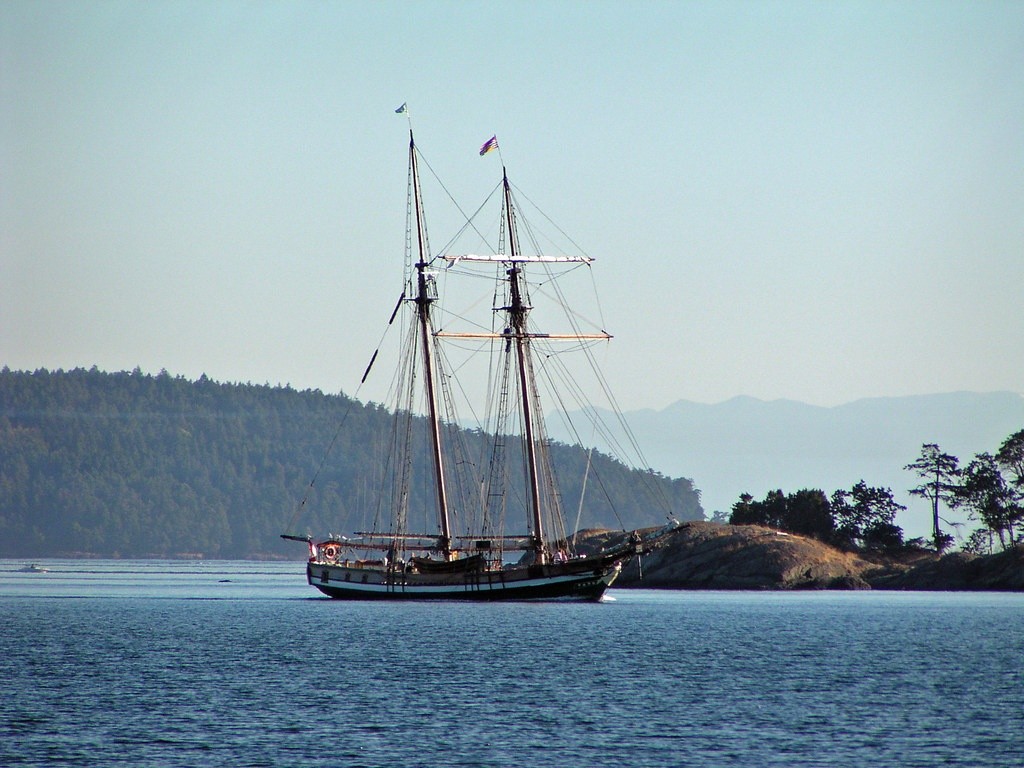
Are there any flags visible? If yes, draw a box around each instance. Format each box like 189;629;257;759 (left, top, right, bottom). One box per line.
395;103;406;113
479;136;497;157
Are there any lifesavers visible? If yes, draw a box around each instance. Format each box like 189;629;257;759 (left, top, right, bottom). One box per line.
325;546;336;559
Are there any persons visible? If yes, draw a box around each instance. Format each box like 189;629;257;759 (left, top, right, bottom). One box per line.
386;530;641;574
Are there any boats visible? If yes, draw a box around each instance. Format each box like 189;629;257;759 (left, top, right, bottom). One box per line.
21;566;50;576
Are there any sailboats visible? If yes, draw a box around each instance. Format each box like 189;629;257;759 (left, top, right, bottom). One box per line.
280;109;690;601
